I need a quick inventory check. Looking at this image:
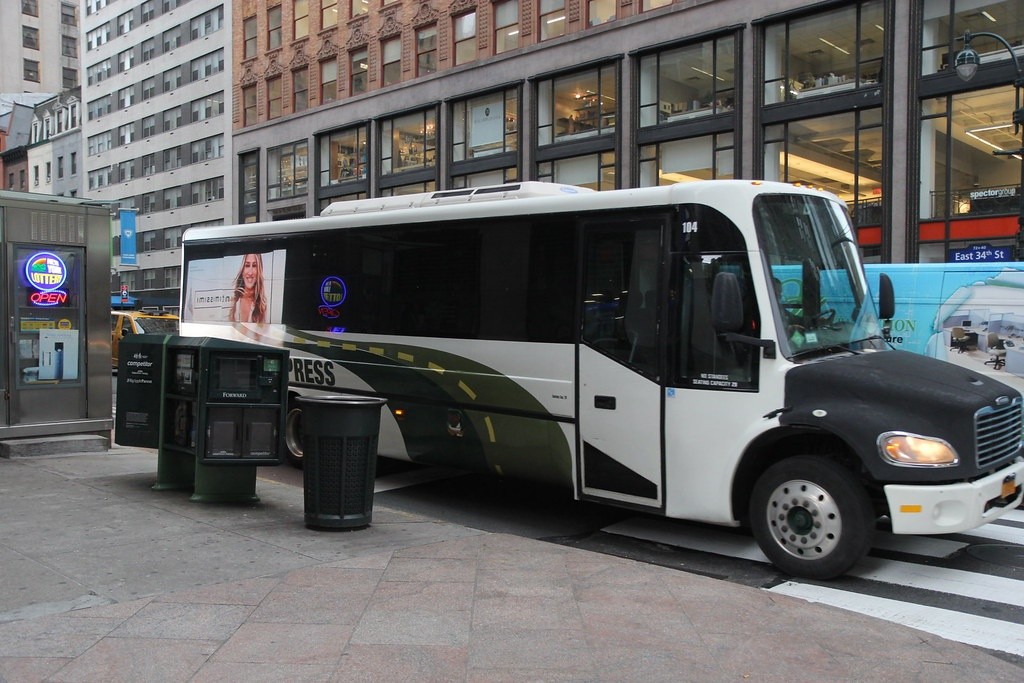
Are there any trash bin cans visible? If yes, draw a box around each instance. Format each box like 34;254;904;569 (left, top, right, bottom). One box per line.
295;394;389;530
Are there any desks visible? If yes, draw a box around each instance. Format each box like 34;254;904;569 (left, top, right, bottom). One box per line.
667;105;729;123
797;80;864;98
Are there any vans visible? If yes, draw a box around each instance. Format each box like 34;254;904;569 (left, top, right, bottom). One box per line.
180;182;1023;580
111;310;178;368
714;259;1023;397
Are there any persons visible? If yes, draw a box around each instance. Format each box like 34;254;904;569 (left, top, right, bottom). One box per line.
772;278;831;336
343;157;347;176
808;73;815;87
229;254;266;323
569;115;574;132
575;117;581;132
724;97;734;107
863;72;875;79
349;157;354;176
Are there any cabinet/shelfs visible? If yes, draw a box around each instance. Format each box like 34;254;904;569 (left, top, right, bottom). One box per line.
574;93;612;132
